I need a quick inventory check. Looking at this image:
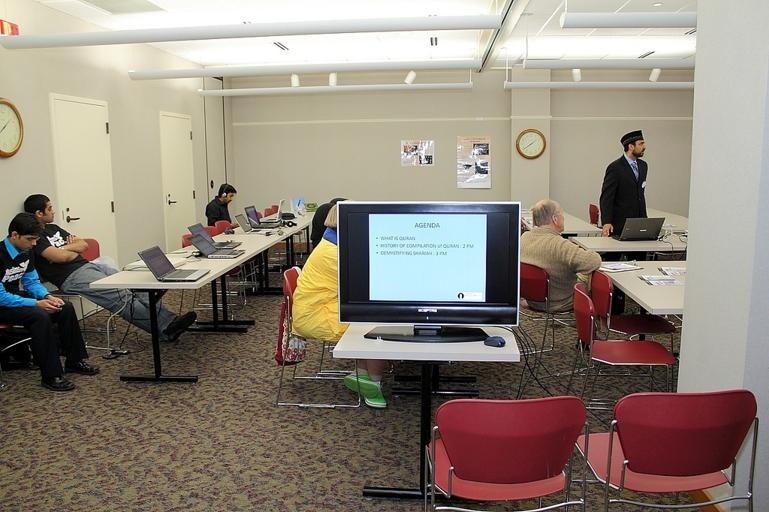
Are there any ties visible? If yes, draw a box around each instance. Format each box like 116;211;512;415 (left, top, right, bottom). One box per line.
631;160;639;181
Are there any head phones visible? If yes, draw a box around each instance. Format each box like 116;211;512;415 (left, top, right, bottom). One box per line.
283;220;297;227
222;184;229;198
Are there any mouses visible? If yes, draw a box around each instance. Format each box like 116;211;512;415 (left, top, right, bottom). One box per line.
484;336;505;348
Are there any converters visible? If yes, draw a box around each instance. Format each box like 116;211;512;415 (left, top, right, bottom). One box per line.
110;349;128;355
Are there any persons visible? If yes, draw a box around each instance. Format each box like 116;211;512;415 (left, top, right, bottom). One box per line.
0;213;98;391
521;199;602;352
205;184;261;281
24;194;197;343
600;130;648;316
292;203;387;409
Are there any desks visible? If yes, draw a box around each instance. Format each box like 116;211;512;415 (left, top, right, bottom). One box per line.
333;322;520;500
598;260;686;340
258;210;316;272
88;253;249;382
171;233;290;326
522;208;604;239
646;207;688;235
567;236;687;314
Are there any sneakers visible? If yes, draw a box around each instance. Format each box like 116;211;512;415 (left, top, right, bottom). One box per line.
344;374;379;398
364;384;387;409
163;311;197;342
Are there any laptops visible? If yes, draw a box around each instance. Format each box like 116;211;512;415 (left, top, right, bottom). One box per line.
611;217;665;241
234;214;272;235
259;199;285;223
244;205;280;229
187;222;242;249
137;246;210;281
187;234;245;259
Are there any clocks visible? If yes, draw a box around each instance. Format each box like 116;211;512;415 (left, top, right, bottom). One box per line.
0;98;24;158
516;128;547;160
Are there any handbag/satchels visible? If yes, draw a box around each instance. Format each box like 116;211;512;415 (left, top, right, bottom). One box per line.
275;302;306;366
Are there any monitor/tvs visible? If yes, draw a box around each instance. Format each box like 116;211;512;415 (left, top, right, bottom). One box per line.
336;200;521;342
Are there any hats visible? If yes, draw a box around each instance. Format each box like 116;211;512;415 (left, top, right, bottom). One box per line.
620;130;644;147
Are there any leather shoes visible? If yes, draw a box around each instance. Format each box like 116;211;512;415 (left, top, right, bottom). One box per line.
65;359;99;375
41;375;76;391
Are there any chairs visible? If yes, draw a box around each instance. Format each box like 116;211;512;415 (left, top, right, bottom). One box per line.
275;267;363;409
178;205;278;329
424;394;590;512
568;270;675;389
573;388;759;511
565;282;678;410
521;262;594;382
589;204;603;229
0;238;119;368
